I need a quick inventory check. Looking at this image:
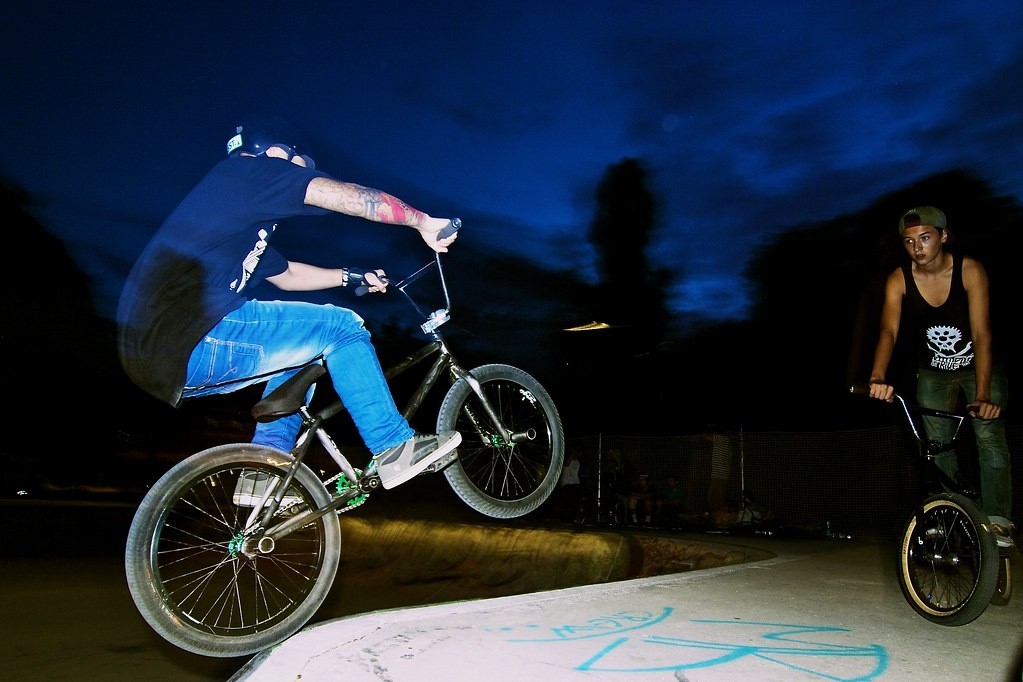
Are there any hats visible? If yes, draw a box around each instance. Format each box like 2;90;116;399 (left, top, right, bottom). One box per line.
897;205;951;236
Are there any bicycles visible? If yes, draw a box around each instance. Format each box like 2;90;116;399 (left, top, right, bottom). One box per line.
847;384;1014;627
124;216;565;659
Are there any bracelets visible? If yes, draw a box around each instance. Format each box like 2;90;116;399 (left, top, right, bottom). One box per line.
341;267;350;288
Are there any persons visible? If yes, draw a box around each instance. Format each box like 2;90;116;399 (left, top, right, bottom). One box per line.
539;442;691;531
117;118;462;512
870;209;1017;548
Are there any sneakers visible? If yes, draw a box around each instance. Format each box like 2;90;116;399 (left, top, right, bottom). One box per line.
232;470;305;509
926;521;945;536
990;522;1014;548
371;429;463;489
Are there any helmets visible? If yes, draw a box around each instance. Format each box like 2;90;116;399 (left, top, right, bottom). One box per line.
227;116;317;170
